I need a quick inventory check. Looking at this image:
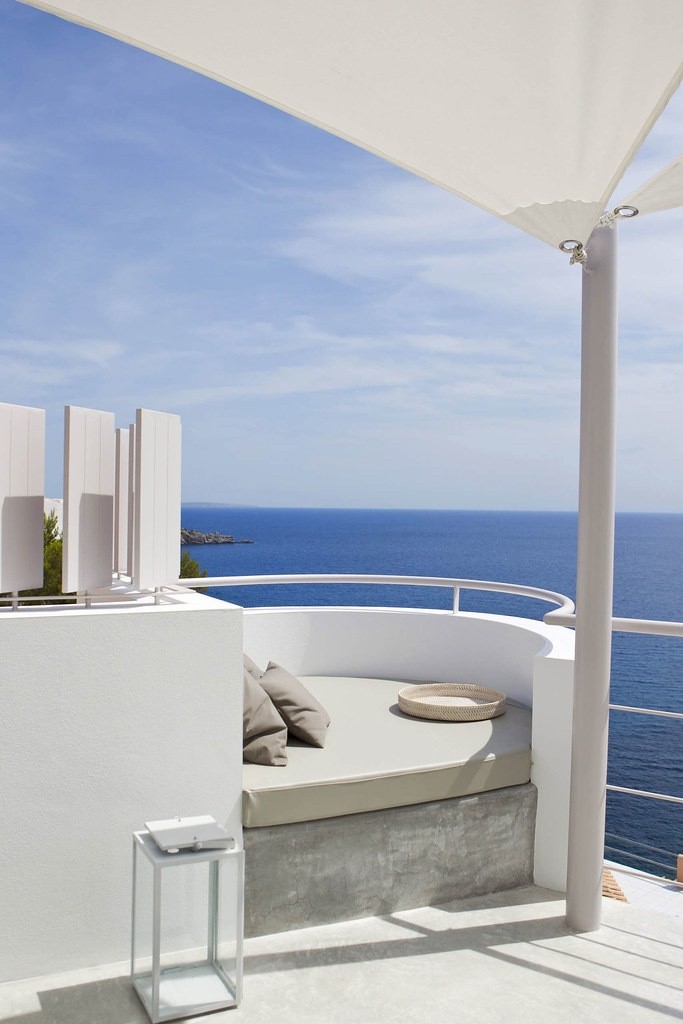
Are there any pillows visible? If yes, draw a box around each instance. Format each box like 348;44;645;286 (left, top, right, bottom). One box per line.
243;653;266;683
243;666;289;766
258;660;333;748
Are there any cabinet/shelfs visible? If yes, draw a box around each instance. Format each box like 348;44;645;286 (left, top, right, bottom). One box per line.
130;829;245;1024
